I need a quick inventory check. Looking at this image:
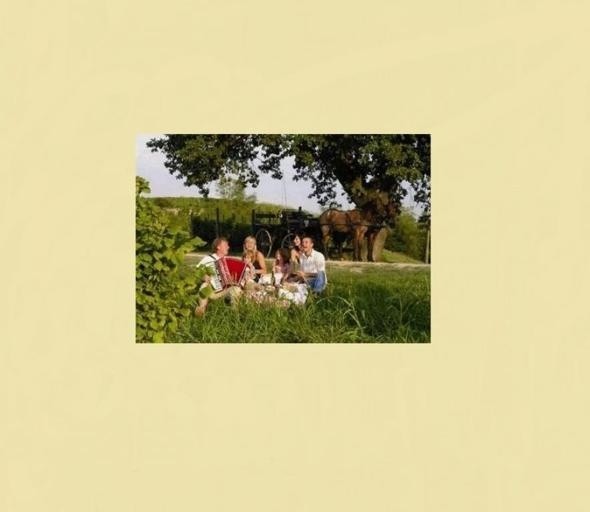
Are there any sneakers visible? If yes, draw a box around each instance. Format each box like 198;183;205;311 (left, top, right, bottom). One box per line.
311;291;318;303
195;306;205;318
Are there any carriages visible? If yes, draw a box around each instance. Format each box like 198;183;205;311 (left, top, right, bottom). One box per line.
251;195;400;263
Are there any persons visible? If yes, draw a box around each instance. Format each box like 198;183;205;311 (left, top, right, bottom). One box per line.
195;237;242;317
299;237;328;292
290;234;301;264
272;248;305;284
242;236;266;283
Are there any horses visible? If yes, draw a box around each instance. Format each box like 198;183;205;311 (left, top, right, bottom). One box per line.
318;196;397;263
